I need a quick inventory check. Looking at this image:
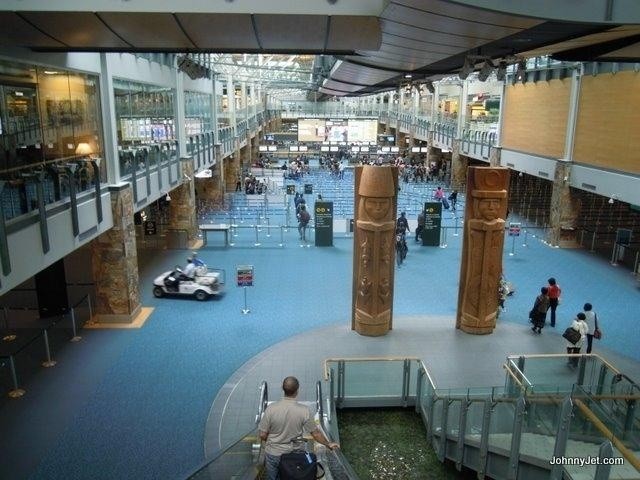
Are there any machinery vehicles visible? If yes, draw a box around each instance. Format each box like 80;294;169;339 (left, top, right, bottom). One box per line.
153;262;226;301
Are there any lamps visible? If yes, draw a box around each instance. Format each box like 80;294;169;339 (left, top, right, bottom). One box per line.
160;188;171;202
426;80;435;92
608;193;616;204
459;53;527;81
519;169;526;176
75;140;93;154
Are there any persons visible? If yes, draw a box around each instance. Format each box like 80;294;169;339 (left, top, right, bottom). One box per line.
498;276;506;313
315;194;322;203
434;187;443;202
170;258;195;285
532;287;550;334
319;146;449;183
235;137;311;239
396;211;425;271
547;278;561;328
448;189;458;211
564;312;589;372
258;377;341;479
191;251;205;267
583;303;598;353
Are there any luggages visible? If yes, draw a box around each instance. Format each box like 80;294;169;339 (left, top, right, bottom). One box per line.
278;439;317;480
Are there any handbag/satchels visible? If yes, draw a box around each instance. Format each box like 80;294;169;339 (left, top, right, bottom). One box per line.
563;328;582;345
594;329;601;339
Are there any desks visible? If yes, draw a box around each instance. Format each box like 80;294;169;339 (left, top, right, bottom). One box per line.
198;224;231;246
